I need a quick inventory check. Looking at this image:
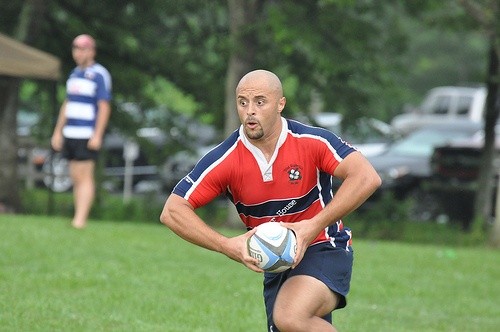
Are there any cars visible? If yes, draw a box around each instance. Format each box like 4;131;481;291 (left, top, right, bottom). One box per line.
359;126;498;224
14;93;188;193
391;86;490;142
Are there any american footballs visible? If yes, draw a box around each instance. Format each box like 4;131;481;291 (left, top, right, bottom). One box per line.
247;221;296;274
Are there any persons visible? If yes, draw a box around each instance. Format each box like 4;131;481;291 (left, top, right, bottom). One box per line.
159;71;382;331
50;34;113;232
